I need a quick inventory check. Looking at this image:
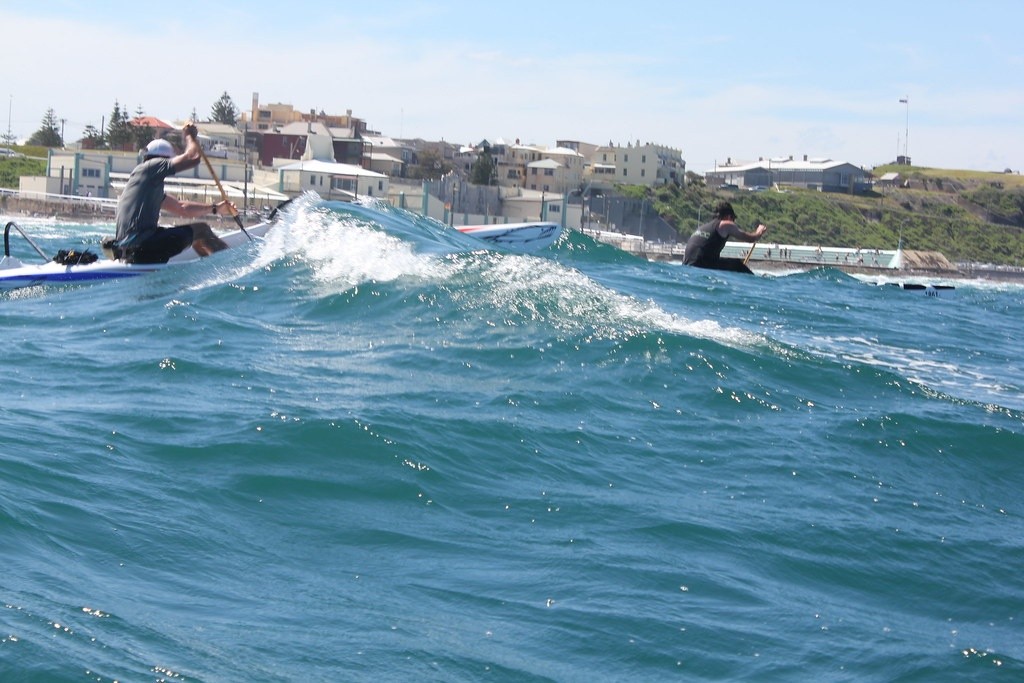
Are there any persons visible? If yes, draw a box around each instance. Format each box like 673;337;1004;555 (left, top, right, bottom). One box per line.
115;138;231;265
681;201;755;277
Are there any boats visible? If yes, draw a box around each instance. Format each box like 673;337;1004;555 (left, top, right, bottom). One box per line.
649;257;955;300
451;221;563;253
0;199;298;289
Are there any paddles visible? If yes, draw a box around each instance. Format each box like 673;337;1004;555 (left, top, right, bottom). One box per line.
743;241;756;264
194;138;255;244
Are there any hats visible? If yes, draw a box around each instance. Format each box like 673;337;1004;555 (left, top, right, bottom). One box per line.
714;201;738;219
141;138;176;159
